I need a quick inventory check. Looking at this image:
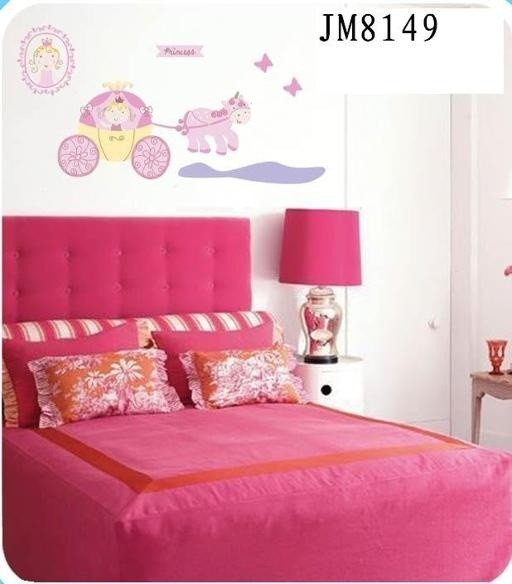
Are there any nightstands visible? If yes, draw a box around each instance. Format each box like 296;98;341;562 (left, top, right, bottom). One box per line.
289;353;365;414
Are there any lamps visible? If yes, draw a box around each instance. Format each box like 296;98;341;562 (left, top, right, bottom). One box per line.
278;208;365;364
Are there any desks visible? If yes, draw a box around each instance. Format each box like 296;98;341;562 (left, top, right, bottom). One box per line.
468;370;512;444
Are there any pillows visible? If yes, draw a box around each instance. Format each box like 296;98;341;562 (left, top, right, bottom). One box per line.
1;315;159;429
131;309;285;409
25;346;187;429
177;340;312;412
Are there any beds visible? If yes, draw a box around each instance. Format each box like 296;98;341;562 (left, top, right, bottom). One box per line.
2;215;512;582
